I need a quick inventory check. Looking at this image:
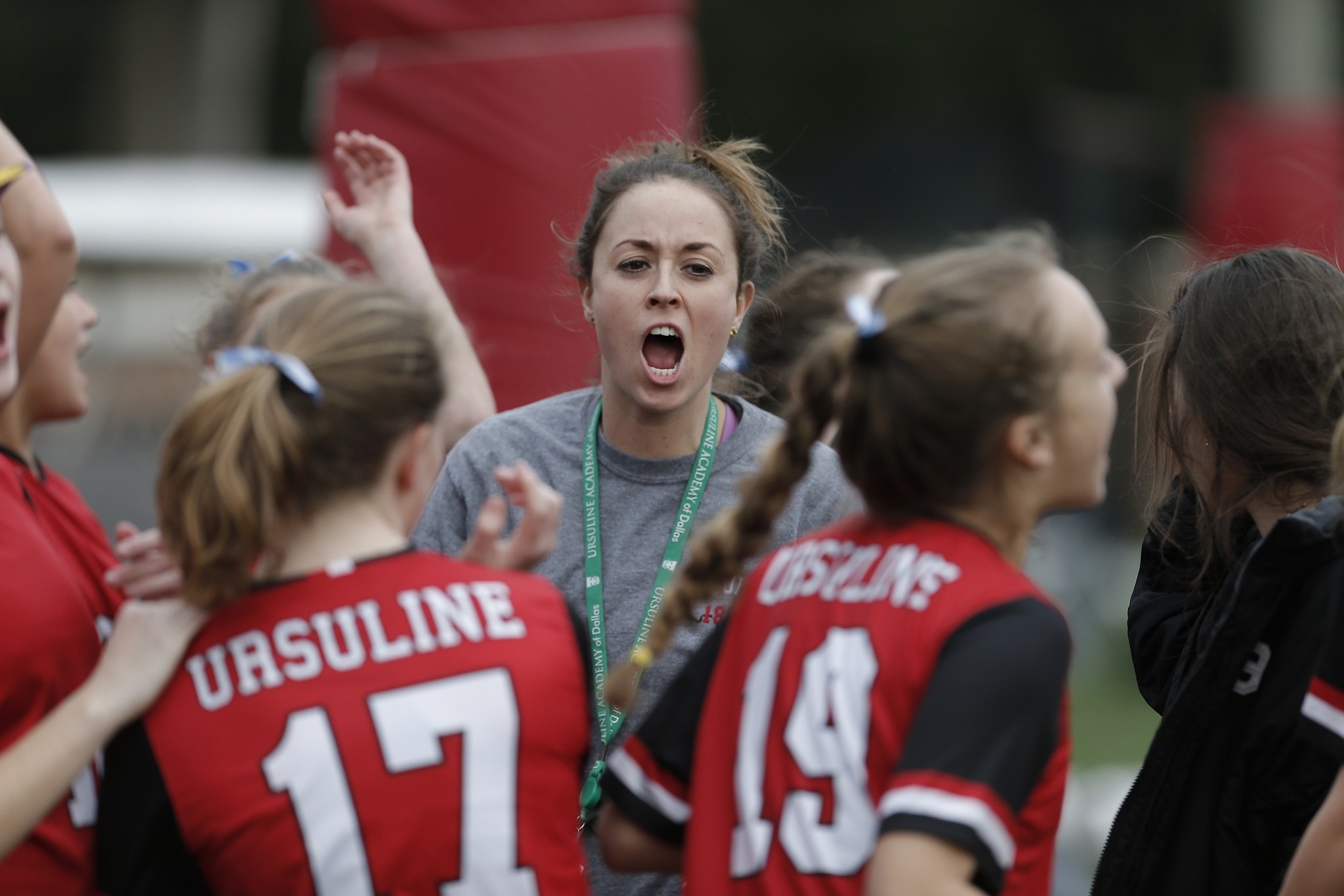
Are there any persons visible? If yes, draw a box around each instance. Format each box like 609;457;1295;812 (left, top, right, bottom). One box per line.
202;130;496;450
748;250;894;423
0;122;215;896
597;234;1128;896
87;277;593;896
1089;247;1344;896
413;146;866;896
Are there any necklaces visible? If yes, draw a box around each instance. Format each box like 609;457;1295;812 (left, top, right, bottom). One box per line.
1287;499;1320;516
